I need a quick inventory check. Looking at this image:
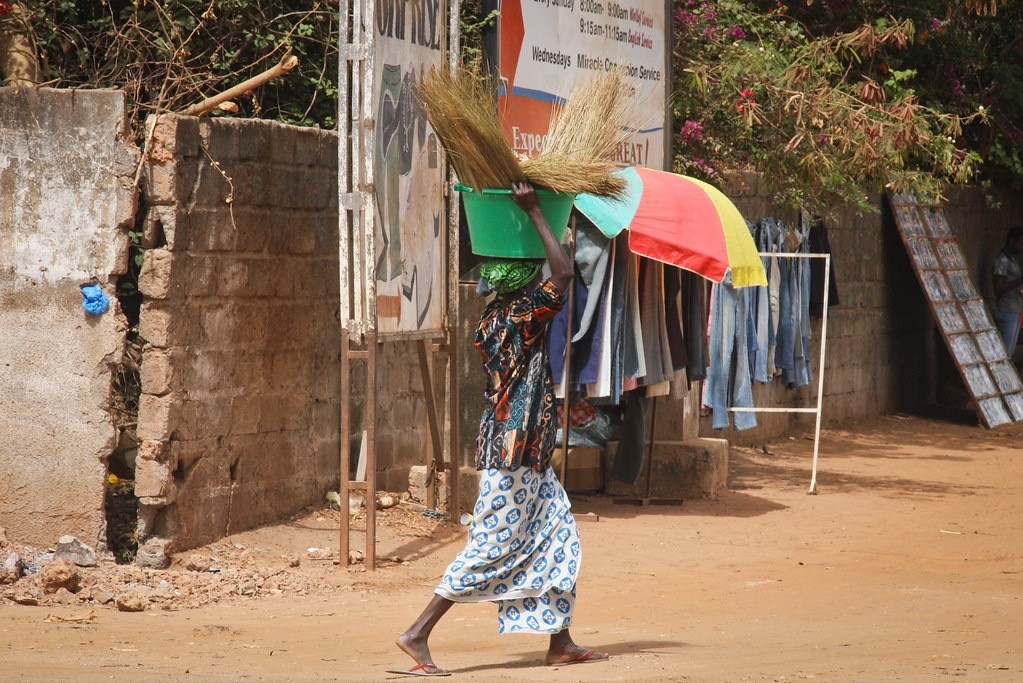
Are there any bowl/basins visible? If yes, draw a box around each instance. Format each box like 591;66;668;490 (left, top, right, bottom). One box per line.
454;182;578;258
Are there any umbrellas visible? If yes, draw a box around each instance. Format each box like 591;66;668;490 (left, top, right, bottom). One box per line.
573;166;768;290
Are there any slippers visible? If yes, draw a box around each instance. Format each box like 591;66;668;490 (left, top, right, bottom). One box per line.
544;646;611;667
385;661;453;677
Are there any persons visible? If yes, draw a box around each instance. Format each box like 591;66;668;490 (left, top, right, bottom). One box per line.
385;181;611;677
